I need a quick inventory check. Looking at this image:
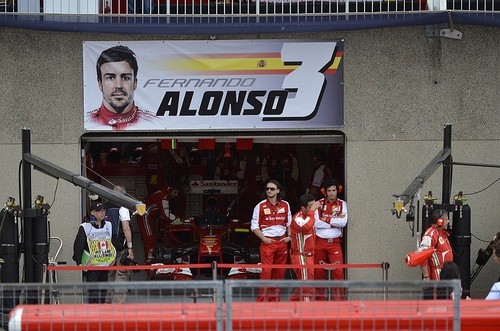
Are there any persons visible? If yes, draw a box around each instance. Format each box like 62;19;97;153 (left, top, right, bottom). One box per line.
306;148;345;200
431;262;472;301
72;203;117;305
291;195;322;302
137;181;188;279
83;46;167;132
311;180;349;303
416;211;456;300
101;184;133;304
250;181;292;303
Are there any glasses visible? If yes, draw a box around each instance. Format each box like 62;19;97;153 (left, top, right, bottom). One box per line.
266;187;279;191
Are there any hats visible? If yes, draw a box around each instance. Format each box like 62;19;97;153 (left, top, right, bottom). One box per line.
90;202;108;211
